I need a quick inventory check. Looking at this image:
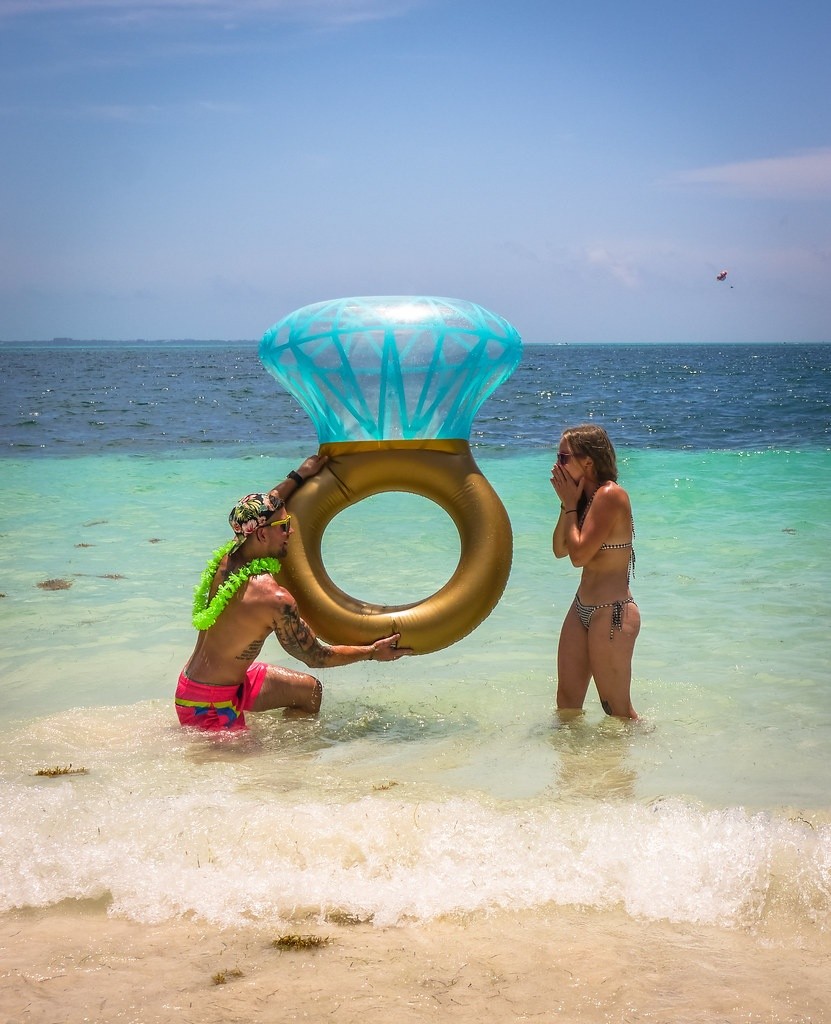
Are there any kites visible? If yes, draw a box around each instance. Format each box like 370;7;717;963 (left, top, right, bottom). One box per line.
717;271;735;288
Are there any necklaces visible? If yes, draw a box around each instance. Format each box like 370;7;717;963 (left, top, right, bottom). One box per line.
191;540;281;631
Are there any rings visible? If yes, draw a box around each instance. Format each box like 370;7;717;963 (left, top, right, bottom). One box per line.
393;656;396;659
252;293;523;656
558;480;561;485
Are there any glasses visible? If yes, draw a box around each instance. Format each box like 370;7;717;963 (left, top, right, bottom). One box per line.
259;515;291;534
557;452;571;465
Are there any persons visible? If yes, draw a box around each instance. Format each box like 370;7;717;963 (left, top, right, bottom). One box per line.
549;424;642;720
174;455;416;736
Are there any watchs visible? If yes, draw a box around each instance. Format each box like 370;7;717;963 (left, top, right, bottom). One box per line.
286;470;304;486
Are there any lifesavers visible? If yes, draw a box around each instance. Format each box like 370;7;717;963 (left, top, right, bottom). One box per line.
253;294;526;658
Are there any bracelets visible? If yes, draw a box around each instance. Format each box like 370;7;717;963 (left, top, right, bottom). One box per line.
561;503;565;510
369;645;376;661
565;510;577;514
